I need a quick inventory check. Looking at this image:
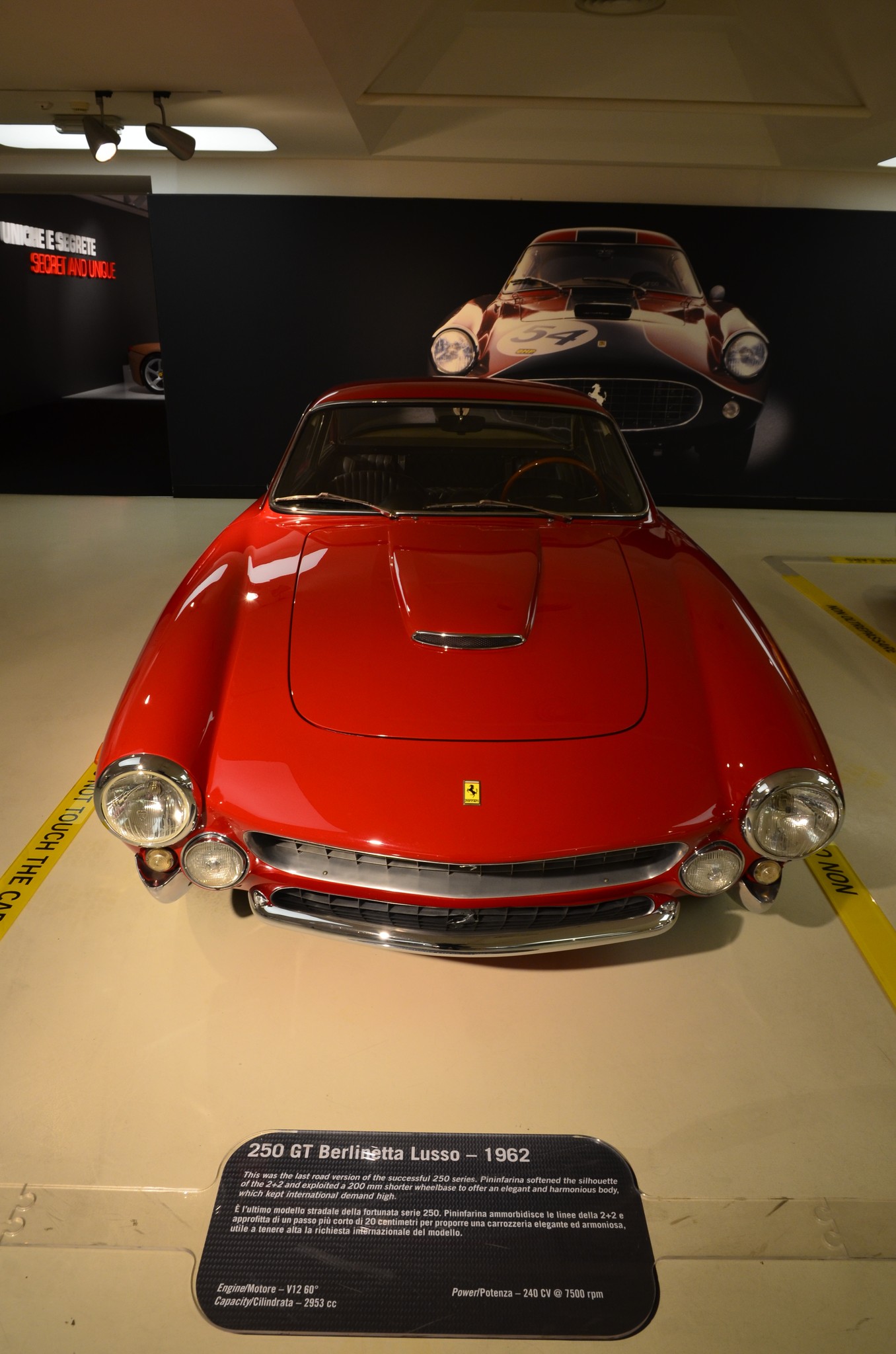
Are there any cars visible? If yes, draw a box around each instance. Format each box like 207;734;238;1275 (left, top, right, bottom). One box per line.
91;378;848;962
424;226;773;471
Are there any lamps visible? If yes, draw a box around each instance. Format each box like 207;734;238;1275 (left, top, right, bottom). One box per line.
144;92;196;161
81;90;123;162
